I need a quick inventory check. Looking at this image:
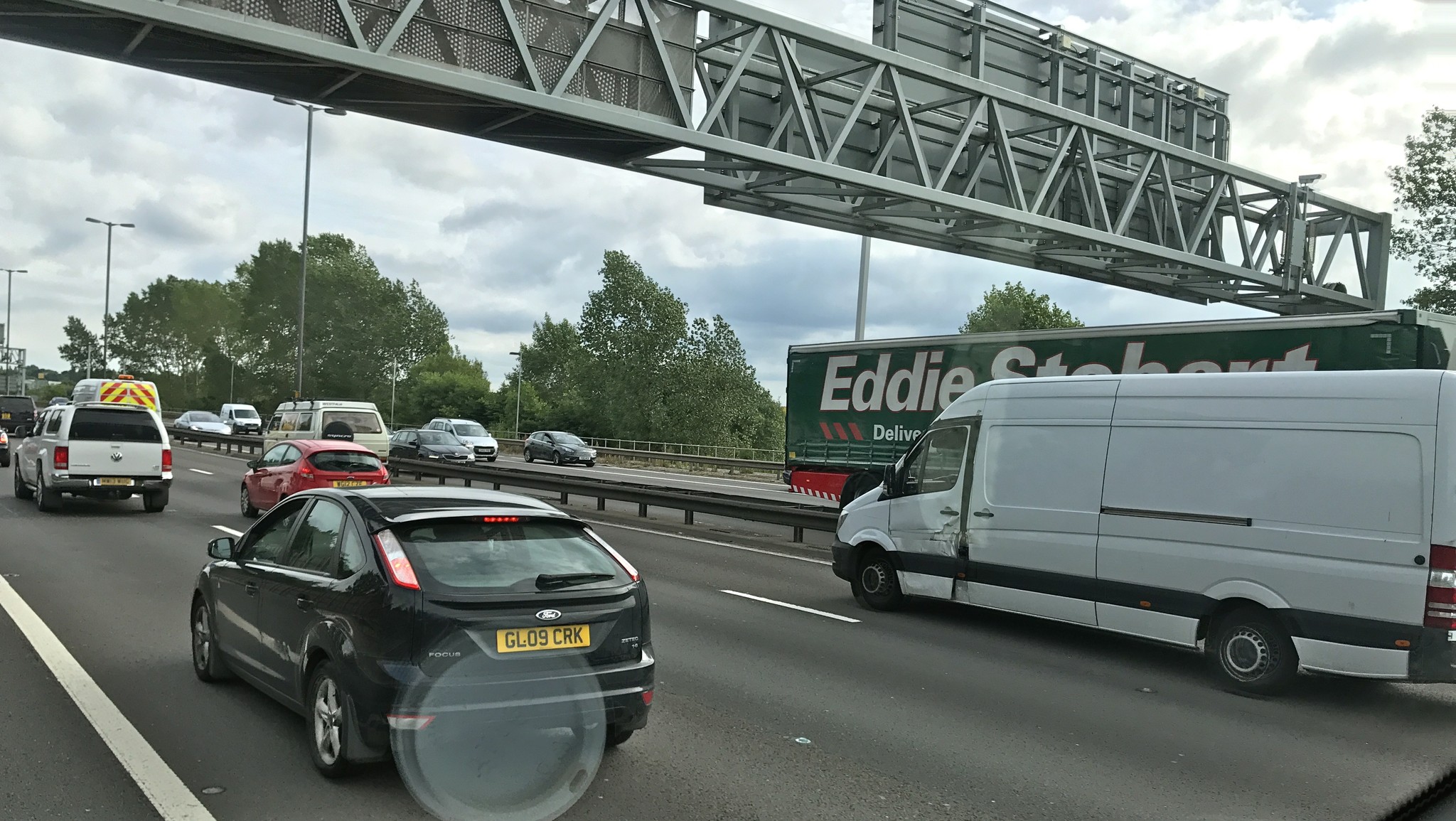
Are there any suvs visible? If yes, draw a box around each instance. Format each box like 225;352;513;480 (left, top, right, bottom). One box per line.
14;401;173;513
0;395;38;434
428;417;499;462
261;391;389;471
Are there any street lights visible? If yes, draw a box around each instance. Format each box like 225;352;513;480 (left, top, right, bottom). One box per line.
271;95;347;398
85;217;136;379
510;352;523;440
391;357;405;432
0;268;28;395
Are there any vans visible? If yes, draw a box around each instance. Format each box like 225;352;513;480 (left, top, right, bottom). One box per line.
220;403;263;435
66;375;162;420
829;370;1455;699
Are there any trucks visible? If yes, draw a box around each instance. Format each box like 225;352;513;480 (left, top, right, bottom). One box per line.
784;304;1455;500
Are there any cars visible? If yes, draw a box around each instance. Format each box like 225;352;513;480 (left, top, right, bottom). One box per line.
523;430;597;468
48;397;69;406
240;439;391;518
0;424;11;467
384;423;475;477
174;410;232;442
189;483;656;779
